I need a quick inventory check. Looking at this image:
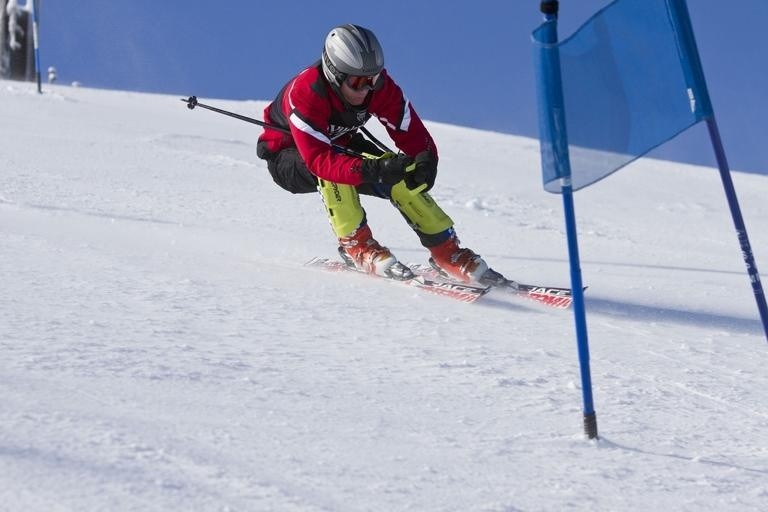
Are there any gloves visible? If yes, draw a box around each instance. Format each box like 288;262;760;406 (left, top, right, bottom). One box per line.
360;154;414;185
404;151;437;193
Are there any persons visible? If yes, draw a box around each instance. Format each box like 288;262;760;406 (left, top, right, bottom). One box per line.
253;21;492;290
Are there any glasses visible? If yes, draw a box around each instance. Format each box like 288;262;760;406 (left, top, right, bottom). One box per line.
345;73;381;90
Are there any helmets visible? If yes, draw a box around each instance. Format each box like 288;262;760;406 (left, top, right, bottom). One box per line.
321;24;384;89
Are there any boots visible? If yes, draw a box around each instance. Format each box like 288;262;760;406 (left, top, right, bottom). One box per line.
337;223;396;277
427;233;488;283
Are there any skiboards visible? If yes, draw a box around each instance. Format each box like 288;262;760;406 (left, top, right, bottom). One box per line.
304;257;588;309
